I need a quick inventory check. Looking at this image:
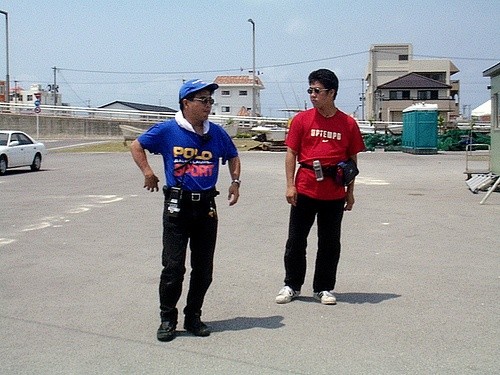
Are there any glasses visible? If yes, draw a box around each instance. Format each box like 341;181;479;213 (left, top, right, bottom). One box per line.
191;97;216;104
308;88;329;93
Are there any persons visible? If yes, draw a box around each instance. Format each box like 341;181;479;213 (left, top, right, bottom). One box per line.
129;78;241;341
275;69;366;305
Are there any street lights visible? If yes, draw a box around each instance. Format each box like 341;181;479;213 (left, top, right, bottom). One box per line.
0;9;11;113
361;78;365;121
247;18;256;124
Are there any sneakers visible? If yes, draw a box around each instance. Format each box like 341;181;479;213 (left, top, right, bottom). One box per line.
184;316;210;336
157;321;178;342
276;286;300;305
313;291;336;305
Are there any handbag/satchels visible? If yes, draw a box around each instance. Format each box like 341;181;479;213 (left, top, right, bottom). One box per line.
298;159;360;185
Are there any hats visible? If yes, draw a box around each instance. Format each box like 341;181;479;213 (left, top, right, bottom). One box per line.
179;79;218;103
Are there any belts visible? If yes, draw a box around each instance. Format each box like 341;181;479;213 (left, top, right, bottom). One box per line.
162;186;219;201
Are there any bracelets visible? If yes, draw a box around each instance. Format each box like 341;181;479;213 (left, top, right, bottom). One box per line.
232;179;240;184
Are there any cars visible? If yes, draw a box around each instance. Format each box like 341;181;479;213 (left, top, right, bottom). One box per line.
0;130;45;175
